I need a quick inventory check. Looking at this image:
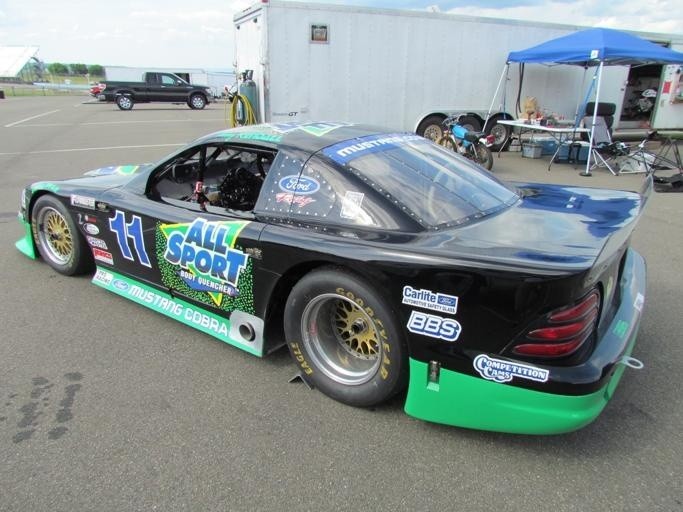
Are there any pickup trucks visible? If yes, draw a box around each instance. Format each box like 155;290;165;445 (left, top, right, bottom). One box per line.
90;71;214;110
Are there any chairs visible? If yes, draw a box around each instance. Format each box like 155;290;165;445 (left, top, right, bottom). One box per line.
583;114;634;178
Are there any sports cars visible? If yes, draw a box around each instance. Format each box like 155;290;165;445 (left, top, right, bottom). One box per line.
15;121;655;435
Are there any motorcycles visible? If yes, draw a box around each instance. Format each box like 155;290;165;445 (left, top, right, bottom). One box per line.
436;114;495;170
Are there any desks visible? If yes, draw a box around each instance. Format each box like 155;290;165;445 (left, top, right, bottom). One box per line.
496;118;592;170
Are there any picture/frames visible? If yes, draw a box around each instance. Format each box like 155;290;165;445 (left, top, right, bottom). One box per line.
310;24;328;43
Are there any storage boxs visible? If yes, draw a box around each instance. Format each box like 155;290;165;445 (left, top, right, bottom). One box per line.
507;134;590;164
